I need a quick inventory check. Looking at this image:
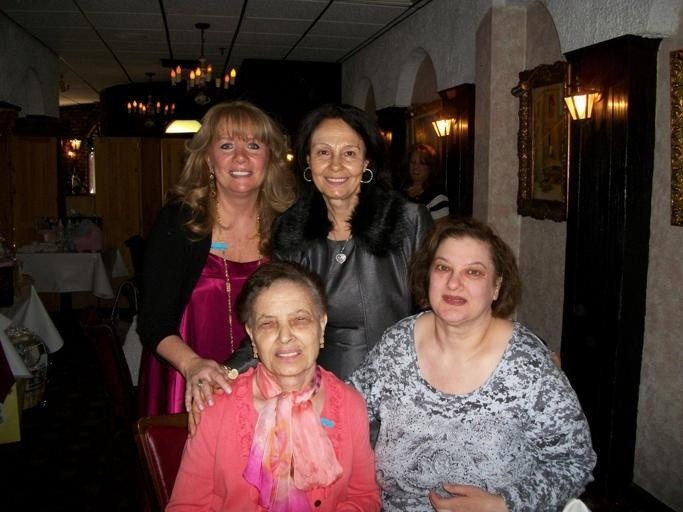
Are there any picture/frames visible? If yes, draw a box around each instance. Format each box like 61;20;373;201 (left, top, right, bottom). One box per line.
406;99;444;149
511;61;568;222
669;49;683;226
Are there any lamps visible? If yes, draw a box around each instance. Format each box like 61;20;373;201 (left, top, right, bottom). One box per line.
169;22;237;90
431;111;458;137
127;72;176;119
562;80;605;121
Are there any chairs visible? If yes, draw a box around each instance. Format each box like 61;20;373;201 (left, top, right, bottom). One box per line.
131;413;187;512
95;274;139;320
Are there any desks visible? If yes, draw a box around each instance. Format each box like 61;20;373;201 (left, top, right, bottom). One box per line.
15;242;115;300
0;284;64;380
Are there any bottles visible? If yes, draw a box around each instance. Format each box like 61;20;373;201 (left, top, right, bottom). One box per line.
64;220;72;246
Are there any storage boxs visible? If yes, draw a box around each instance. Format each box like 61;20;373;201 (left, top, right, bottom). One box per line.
0;379;24;444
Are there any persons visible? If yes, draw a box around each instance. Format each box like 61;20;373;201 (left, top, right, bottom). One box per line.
165;260;385;512
403;144;450;221
272;102;435;383
344;218;598;512
137;101;298;439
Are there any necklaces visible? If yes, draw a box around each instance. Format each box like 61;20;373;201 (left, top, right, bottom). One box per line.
336;237;349;263
215;202;261;354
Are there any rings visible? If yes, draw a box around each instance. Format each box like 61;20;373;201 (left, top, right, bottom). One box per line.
198;380;202;387
